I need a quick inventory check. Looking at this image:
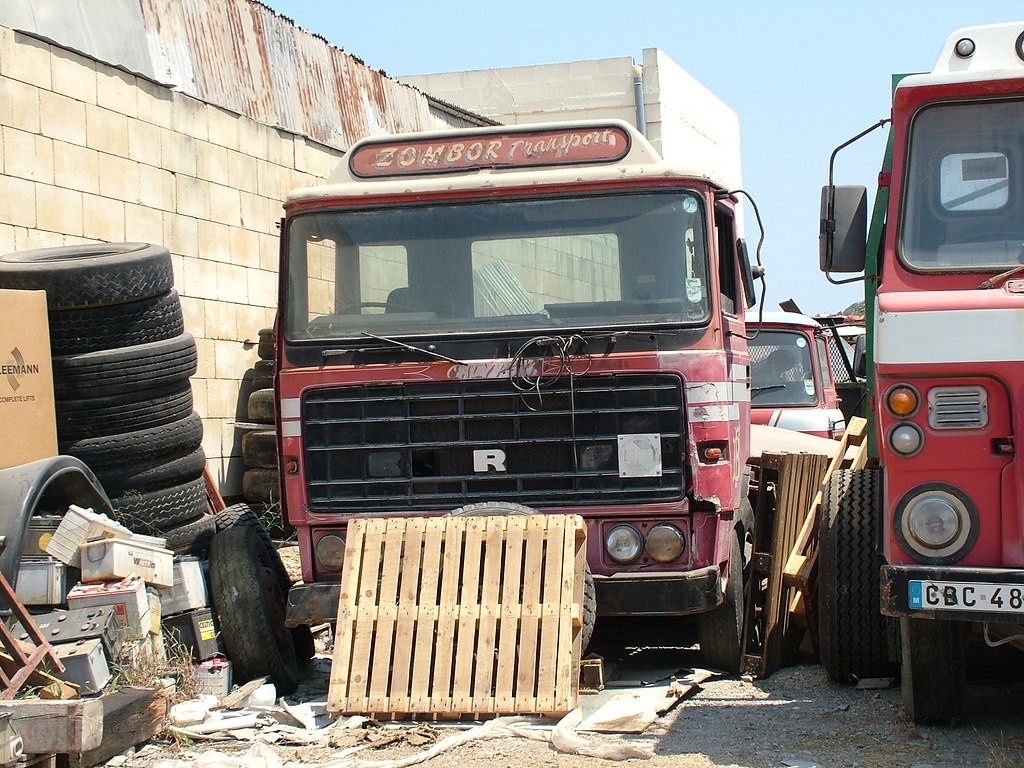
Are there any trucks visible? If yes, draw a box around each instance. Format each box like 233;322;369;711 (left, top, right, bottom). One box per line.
272;122;761;676
732;311;844;489
810;23;1024;726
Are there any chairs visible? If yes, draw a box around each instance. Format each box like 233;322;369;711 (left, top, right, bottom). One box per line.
385;287;445;313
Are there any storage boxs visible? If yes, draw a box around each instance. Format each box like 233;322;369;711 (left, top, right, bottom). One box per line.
14;503;236;703
